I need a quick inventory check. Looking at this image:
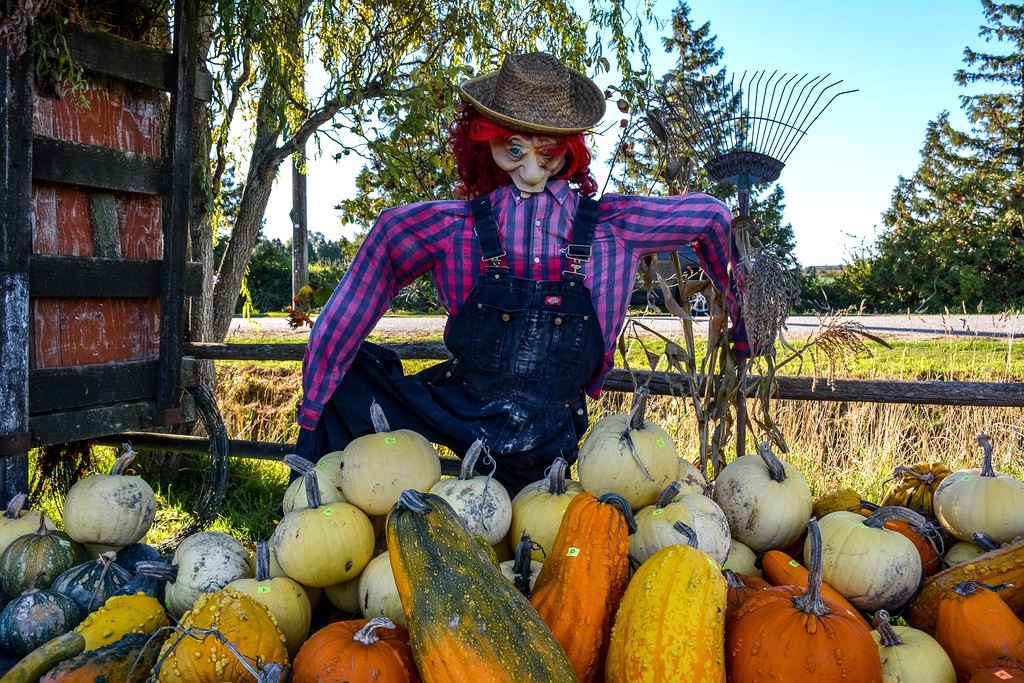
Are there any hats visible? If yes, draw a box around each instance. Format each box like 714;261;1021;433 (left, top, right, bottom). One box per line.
459;52;606;136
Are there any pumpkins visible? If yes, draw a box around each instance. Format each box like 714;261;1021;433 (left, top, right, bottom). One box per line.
0;387;1023;683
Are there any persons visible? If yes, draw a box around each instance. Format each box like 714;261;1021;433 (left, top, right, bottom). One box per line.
678;263;700;289
278;50;761;534
